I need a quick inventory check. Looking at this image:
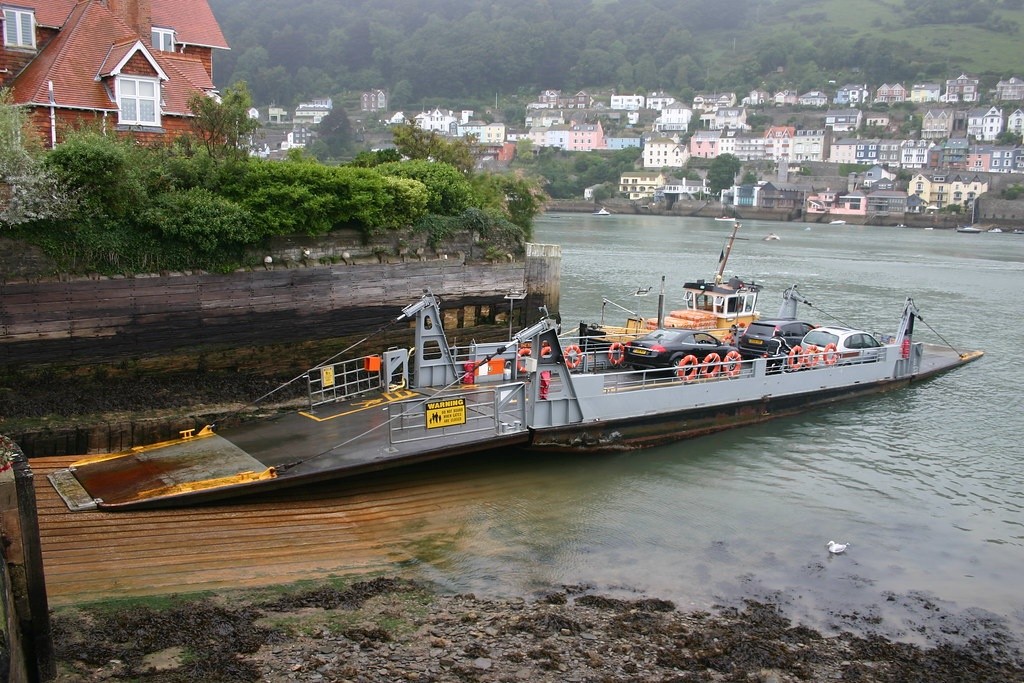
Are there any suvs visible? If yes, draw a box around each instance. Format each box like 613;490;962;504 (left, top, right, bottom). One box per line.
739;317;815;359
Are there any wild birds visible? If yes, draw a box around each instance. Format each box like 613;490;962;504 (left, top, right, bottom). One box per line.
827;541;851;553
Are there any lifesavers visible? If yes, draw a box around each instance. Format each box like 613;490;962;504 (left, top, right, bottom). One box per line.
677;350;741;381
516;347;532;373
563;344;583;369
722;334;735;346
540;345;552;359
788;343;840;369
608;342;626;366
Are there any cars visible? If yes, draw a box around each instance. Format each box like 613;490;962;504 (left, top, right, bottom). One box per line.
624;326;738;377
797;324;890;369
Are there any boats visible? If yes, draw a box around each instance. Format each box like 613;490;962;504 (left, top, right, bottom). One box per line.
956;227;981;234
48;219;985;514
988;228;1002;233
594;208;610;216
715;216;736;221
829;219;846;225
896;223;908;228
